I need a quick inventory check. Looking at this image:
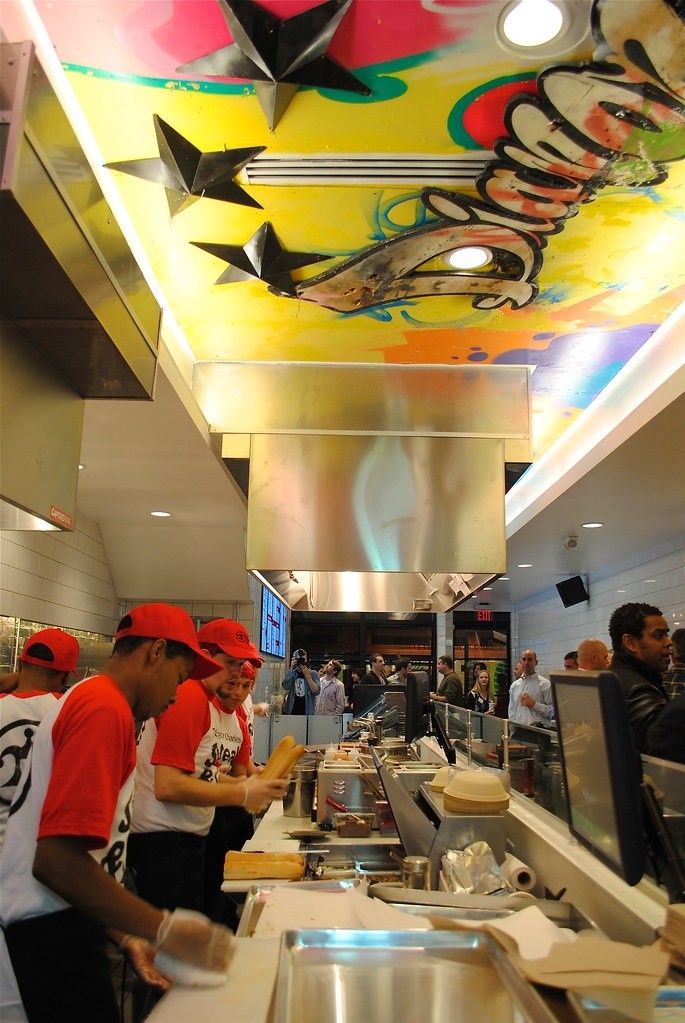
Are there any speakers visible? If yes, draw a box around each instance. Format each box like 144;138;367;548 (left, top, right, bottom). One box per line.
555;576;589;608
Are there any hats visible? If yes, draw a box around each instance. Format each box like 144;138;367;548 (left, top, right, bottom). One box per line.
241;660;254;682
116;602;224;680
197;619;262;669
21;628;83;681
249;642;265;662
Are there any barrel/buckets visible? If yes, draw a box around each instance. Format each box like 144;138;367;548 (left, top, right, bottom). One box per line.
282;765;318;818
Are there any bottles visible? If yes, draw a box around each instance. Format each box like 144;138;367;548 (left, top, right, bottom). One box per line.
335;744;348;760
324;742;337;760
348;746;359;760
402;855;431;891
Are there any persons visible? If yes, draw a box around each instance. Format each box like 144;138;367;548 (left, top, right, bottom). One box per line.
387;658;412;684
508;650;554;798
0;628;80;851
358;653;389;712
563;651;578;670
348;668;366;710
430;655;467;739
577;638;610;671
608;602;685;878
0;602;239;1023
464;663;496;742
311;663;323;677
282;649;321;715
314;659;345;715
126;618;292;1023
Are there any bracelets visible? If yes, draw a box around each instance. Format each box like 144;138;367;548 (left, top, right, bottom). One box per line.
433;696;437;700
240;783;247;806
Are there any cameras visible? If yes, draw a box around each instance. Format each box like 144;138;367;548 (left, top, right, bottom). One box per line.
296;658;305;665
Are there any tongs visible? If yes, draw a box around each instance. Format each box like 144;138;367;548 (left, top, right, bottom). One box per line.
326;796;365;825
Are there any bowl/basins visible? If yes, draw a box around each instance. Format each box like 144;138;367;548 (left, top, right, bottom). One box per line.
429;766;458;793
442;771;510;813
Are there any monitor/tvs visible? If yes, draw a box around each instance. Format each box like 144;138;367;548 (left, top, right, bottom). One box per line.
550;669;685;906
